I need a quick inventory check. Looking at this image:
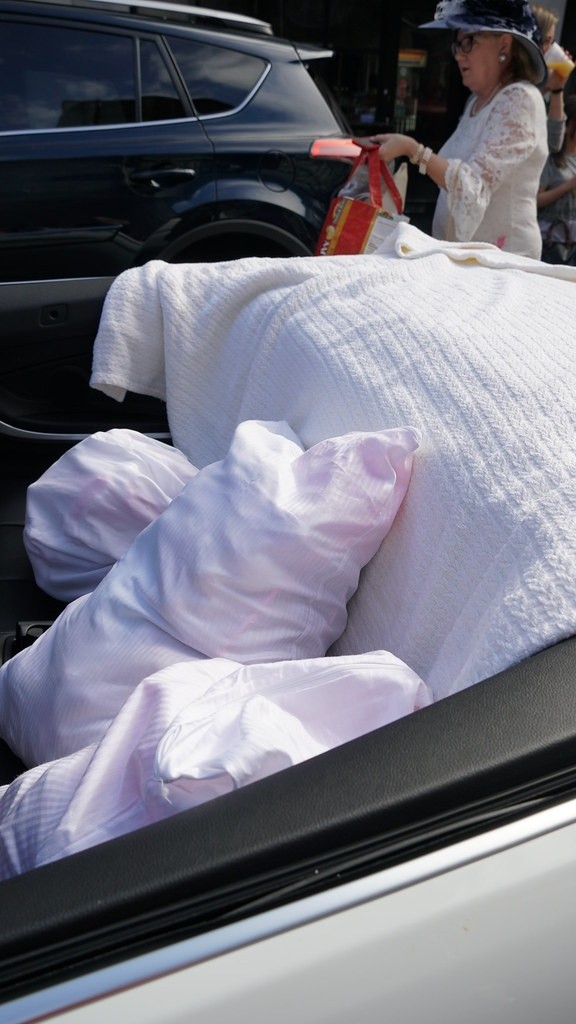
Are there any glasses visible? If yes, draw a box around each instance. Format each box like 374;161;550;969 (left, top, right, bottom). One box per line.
450;32;509;56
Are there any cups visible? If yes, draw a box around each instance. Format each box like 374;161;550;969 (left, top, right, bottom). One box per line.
544;40;575;79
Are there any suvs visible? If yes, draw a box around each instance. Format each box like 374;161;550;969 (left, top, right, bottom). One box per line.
0;1;358;280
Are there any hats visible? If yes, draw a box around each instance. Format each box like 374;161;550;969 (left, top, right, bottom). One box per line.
418;0;548;89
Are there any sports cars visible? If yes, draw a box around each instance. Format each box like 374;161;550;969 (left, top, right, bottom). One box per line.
1;255;573;1024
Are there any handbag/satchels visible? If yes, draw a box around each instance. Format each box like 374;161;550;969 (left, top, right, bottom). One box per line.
540;220;576;267
313;146;407;258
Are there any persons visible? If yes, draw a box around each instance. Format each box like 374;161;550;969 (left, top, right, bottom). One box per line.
536;96;576;267
369;1;550;267
528;6;575;152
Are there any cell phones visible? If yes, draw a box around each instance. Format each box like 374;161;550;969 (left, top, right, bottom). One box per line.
352;136;381;151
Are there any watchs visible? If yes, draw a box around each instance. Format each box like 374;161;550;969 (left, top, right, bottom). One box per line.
548;88;564;95
409;143;424;165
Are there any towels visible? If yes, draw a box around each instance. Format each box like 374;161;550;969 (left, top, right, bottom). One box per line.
90;222;576;712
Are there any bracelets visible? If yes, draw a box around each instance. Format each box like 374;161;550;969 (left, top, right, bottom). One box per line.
418;147;433;175
